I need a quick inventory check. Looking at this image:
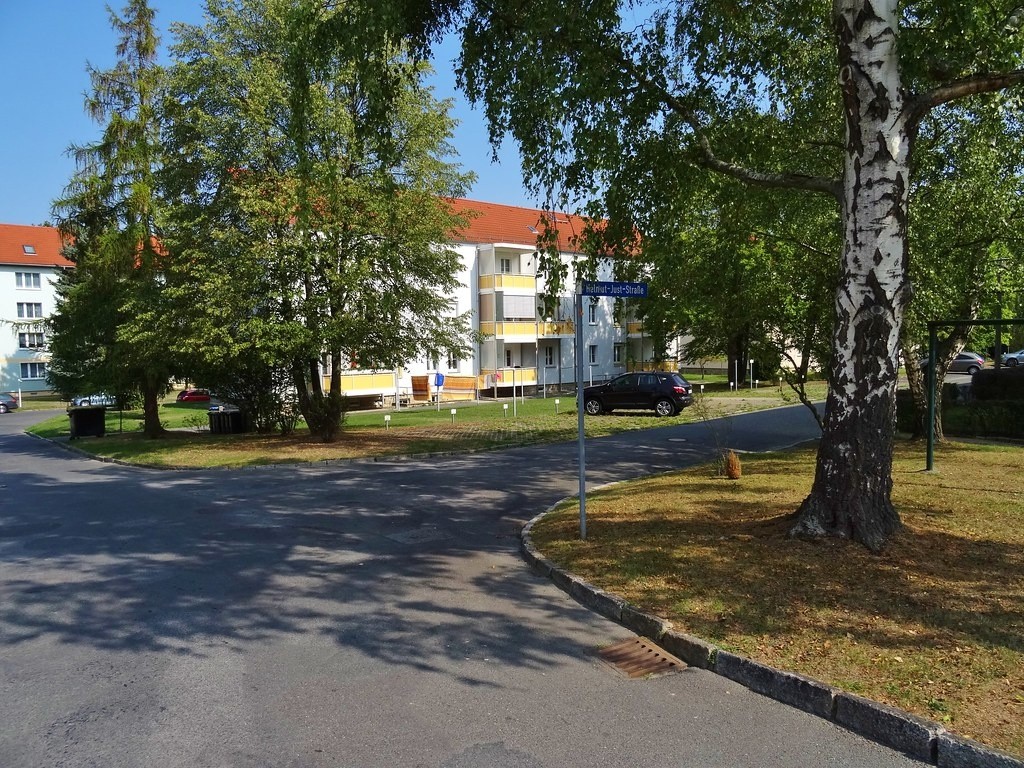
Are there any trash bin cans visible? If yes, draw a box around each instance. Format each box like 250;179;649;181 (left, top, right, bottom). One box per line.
239;403;257;432
65;404;107;441
207;410;243;434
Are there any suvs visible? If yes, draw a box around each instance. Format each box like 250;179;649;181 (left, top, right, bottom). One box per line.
575;370;694;418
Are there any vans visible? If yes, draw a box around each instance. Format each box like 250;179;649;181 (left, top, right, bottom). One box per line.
176;388;210;402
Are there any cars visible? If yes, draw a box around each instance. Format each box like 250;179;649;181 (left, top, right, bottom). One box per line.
1001;349;1024;367
72;390;117;408
0;393;18;414
919;351;985;375
